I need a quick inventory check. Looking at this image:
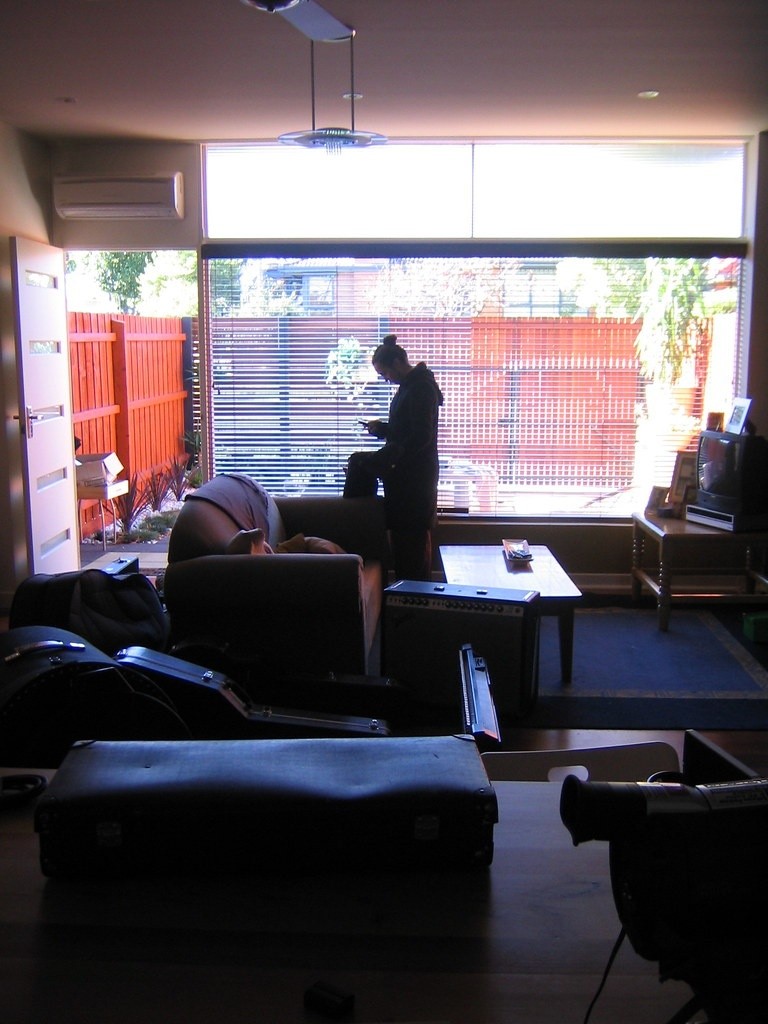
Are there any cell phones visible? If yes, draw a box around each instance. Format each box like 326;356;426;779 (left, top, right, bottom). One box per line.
358;420;369;427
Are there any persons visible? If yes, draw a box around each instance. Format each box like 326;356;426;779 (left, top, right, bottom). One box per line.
367;335;440;580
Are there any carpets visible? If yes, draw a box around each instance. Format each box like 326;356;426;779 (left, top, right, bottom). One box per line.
525;604;768;730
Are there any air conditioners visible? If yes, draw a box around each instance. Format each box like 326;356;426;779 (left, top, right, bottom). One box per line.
53;170;185;221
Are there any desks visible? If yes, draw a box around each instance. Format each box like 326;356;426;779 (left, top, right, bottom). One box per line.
630;514;768;633
76;479;131;551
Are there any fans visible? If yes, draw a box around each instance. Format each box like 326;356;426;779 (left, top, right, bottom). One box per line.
244;0;355;39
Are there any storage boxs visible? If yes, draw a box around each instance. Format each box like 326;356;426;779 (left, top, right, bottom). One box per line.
741;610;768;643
75;451;124;487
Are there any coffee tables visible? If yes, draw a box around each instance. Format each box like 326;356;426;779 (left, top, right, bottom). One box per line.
438;544;582;674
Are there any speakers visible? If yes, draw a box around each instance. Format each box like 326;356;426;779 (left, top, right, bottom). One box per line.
379;580;542;713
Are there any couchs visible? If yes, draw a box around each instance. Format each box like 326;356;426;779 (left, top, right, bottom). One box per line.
163;470;397;681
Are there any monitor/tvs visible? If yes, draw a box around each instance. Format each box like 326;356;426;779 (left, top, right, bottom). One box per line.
695;429;768;514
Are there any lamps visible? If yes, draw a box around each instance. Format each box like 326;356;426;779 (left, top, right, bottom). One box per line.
276;22;390;152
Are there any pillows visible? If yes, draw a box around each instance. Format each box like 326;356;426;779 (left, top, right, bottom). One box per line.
227;528;346;556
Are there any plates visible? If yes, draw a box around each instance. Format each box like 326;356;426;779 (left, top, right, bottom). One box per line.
502;539;534;562
